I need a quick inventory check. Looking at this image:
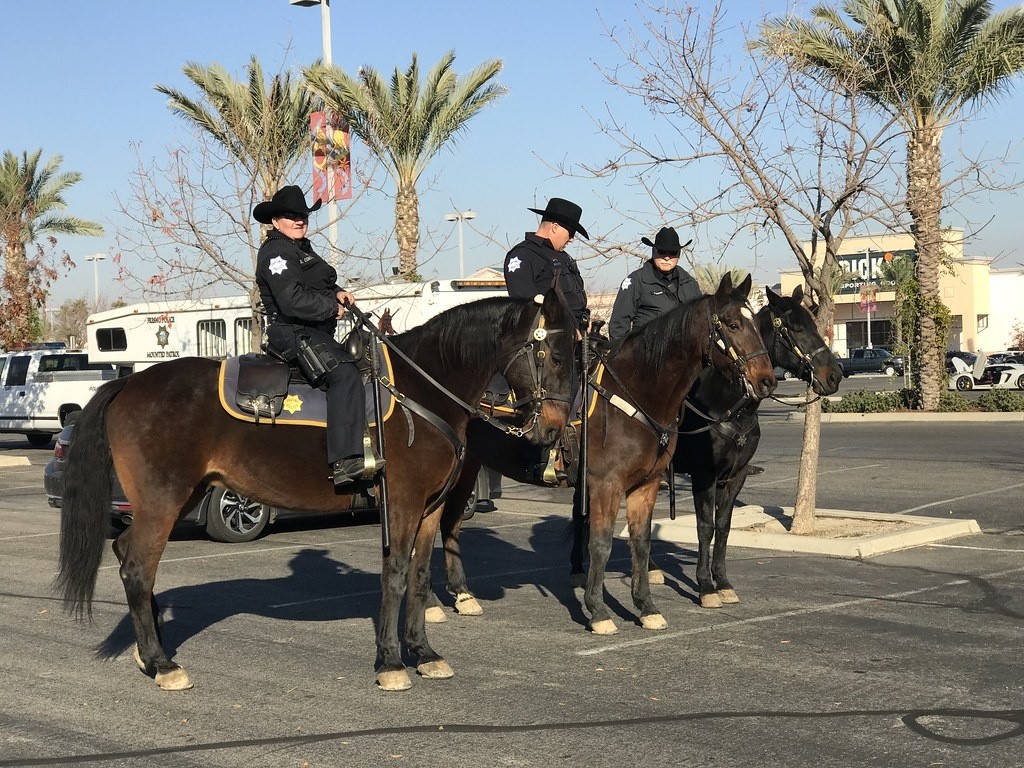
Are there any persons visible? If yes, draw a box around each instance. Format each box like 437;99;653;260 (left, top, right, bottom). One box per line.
252;185;386;486
504;198;590;482
609;226;766;477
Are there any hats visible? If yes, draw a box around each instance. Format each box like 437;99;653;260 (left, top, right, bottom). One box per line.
252;185;323;224
640;226;693;251
526;197;590;241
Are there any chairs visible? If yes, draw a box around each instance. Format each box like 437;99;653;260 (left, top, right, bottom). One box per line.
985;370;1000;384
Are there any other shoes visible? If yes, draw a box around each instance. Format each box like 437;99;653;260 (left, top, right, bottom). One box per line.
327;454;386;486
532;463;569;481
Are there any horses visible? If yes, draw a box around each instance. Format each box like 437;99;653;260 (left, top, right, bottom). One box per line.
46;287;578;691
424;271;778;635
568;284;843;608
